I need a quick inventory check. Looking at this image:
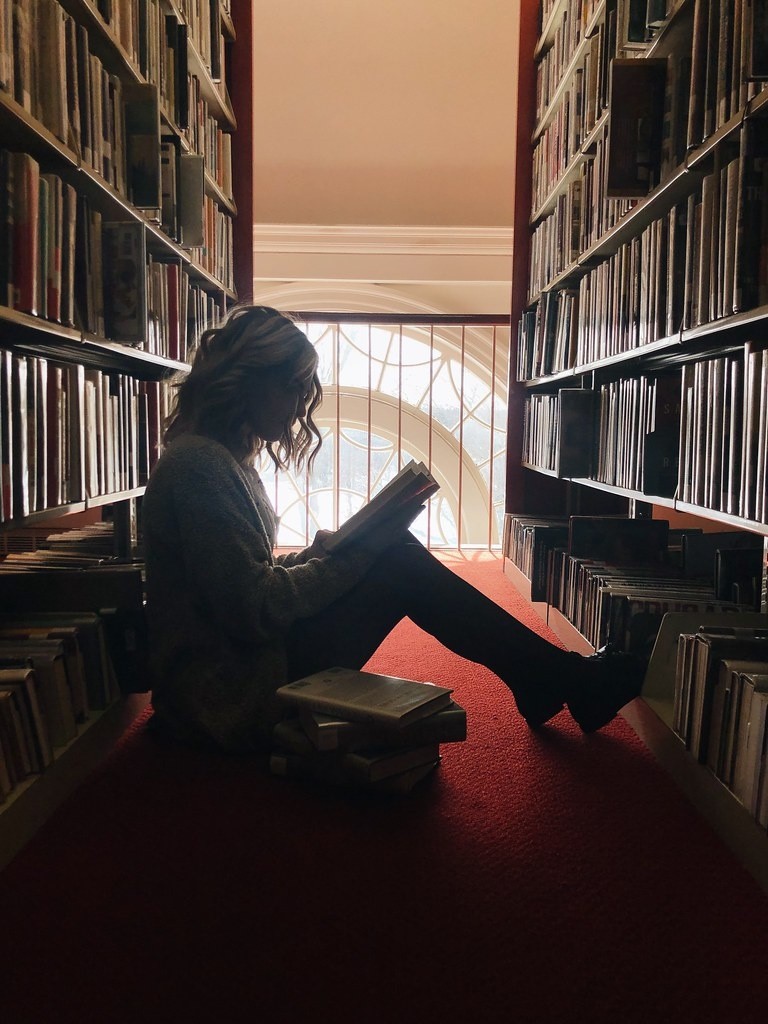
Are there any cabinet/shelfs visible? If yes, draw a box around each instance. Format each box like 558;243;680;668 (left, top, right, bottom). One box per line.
493;0;768;895
0;0;252;873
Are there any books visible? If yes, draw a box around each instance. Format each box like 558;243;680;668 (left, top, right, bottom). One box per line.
322;458;440;553
508;0;766;830
271;668;467;793
1;0;239;804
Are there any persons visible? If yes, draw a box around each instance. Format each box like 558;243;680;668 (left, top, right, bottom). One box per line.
138;305;646;732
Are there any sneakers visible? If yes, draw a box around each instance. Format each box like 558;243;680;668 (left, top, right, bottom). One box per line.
569;635;655;734
516;642;625;729
